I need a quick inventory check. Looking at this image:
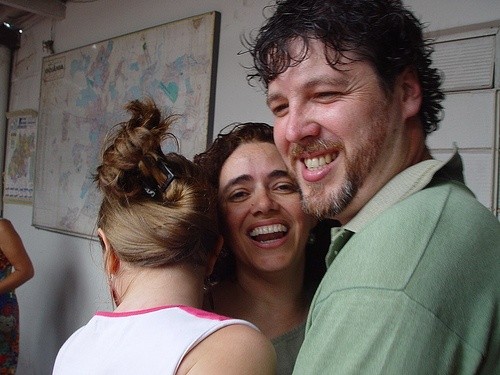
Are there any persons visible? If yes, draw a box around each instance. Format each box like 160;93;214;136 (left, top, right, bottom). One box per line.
52;99;277;375
0;218;34;375
237;0;500;375
193;122;342;375
